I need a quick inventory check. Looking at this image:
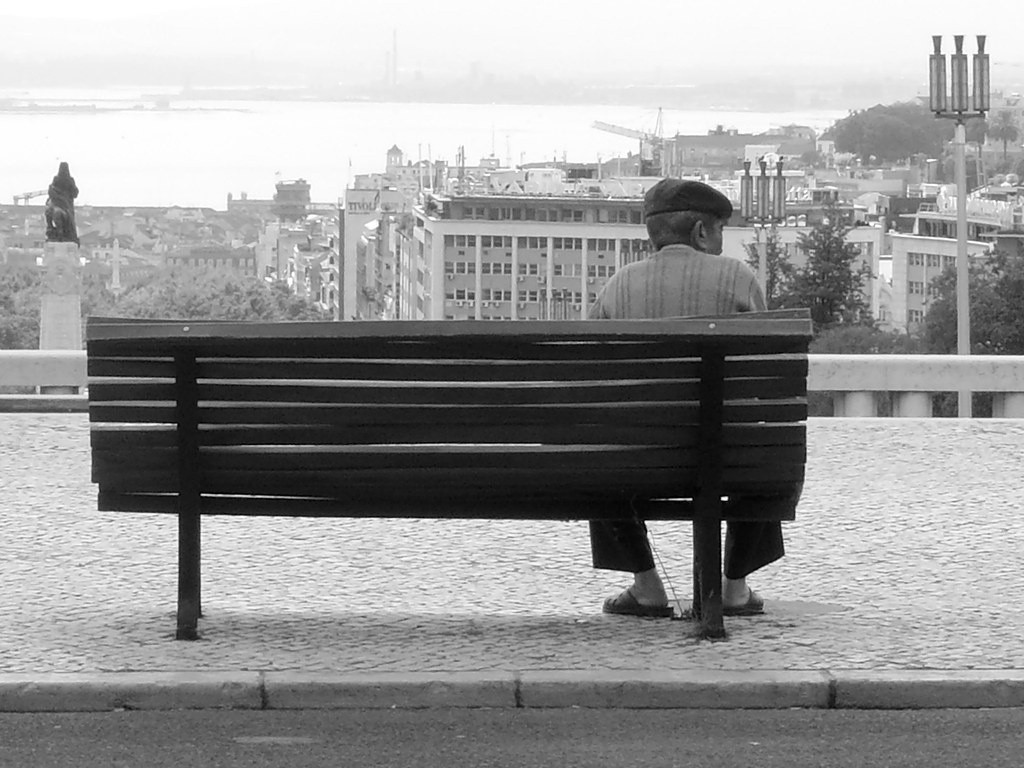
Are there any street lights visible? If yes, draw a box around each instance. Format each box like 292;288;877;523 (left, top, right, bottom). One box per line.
736;156;789;300
929;33;993;417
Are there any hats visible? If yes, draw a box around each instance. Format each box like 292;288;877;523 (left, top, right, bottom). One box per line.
644;178;733;219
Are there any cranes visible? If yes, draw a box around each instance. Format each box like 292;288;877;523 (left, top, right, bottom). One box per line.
589;106;666;151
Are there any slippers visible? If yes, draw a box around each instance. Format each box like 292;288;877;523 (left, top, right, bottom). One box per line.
602;589;674;617
723;585;765;616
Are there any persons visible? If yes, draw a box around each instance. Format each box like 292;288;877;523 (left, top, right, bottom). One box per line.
585;178;786;619
44;161;79;241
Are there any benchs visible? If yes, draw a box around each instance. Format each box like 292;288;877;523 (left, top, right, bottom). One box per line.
83;308;817;643
